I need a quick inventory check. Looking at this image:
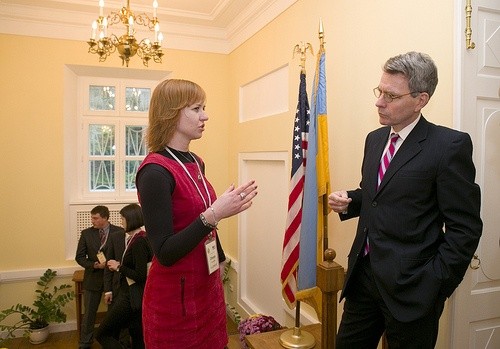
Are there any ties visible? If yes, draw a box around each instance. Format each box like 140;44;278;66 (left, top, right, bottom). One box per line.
100;229;106;247
364;134;399;255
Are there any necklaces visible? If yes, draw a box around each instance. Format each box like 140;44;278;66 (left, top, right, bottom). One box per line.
176;149;201;181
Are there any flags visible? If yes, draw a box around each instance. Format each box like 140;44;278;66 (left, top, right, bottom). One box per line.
295;46;333;323
281;70;310;308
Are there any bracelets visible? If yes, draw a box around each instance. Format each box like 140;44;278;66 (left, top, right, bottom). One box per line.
200;212;215;229
211;206;218;225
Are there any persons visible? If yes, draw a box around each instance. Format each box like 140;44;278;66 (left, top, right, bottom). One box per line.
328;51;483;349
75;206;130;349
94;203;154;349
136;78;258;349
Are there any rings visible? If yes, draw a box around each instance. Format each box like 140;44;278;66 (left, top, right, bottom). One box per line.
240;192;246;199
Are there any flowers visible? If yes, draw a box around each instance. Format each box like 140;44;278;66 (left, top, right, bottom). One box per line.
239;313;285;346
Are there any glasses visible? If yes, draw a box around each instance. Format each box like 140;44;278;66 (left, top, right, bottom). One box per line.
373;87;428;102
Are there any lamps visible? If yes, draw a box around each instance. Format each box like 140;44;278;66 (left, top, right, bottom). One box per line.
88;0;164;69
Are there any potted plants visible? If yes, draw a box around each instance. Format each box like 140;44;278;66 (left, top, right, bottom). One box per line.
0;267;74;349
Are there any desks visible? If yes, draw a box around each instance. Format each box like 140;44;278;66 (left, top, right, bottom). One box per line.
72;269;108;343
